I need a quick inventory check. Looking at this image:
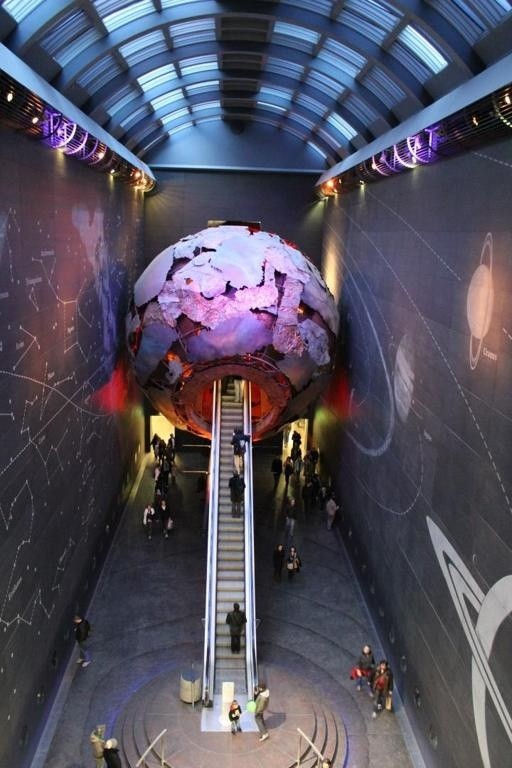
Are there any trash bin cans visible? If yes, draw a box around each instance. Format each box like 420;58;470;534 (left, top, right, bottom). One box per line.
179;675;202;704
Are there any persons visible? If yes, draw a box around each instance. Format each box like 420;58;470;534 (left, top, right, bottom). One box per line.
228;470;246;518
230;428;250;475
253;683;270;742
371;660;394;718
234;377;246;403
228;701;242;734
103;737;121;768
90;724;105;768
271;431;340;581
140;433;176;542
225;603;247;653
197;474;206;503
352;645;375;698
72;615;93;666
